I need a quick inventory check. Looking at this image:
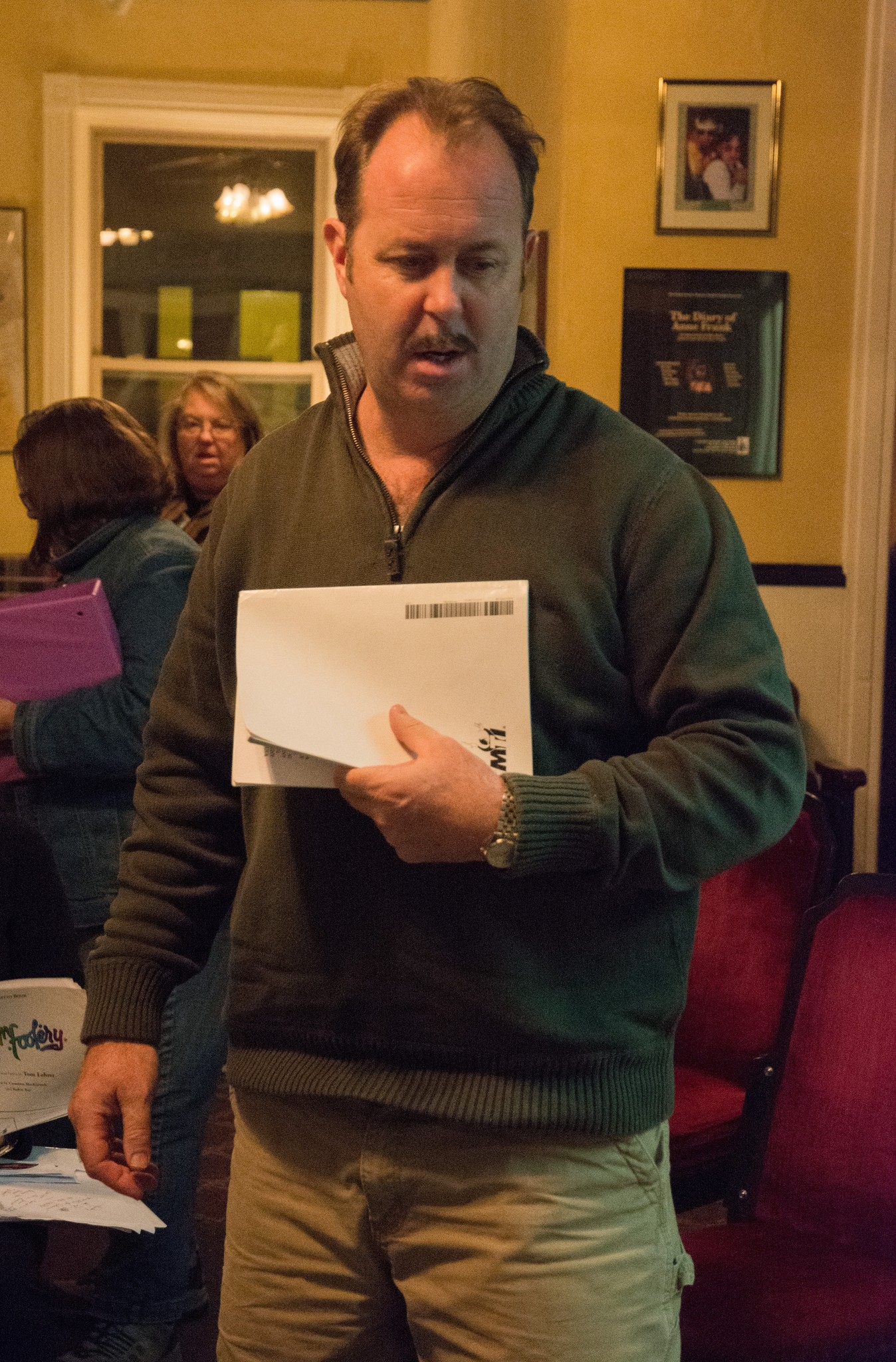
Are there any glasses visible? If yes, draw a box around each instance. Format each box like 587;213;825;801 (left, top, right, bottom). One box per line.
175;414;243;434
693;129;716;135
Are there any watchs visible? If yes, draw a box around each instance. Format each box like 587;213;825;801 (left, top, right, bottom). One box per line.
478;775;518;870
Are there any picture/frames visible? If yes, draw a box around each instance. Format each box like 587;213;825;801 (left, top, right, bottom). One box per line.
653;76;786;237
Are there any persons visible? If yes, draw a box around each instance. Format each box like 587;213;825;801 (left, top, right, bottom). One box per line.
701;127;749;201
2;400;244;1356
71;74;806;1362
685;107;731;187
143;368;266;546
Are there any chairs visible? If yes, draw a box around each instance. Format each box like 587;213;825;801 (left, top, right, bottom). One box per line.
668;790;836;1212
677;871;896;1362
